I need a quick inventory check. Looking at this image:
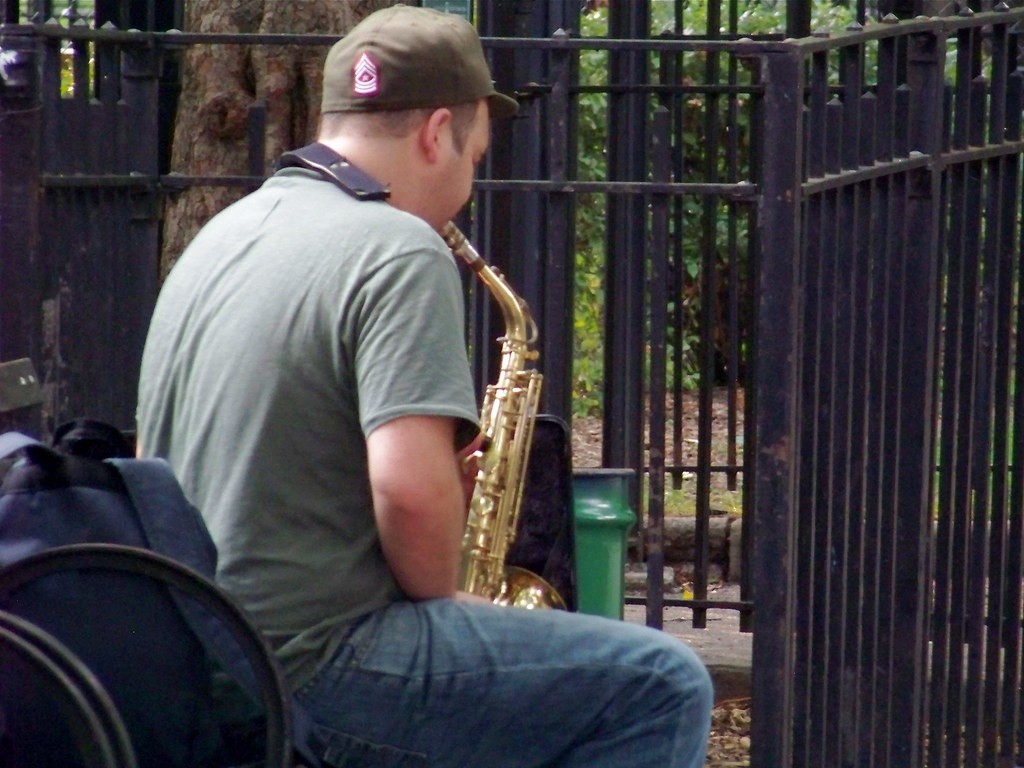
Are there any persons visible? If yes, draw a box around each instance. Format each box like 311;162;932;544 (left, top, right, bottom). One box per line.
139;5;714;768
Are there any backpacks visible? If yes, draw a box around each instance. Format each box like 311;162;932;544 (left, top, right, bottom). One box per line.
0;424;325;768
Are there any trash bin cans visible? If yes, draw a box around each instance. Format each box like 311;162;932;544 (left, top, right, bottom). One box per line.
575;466;636;621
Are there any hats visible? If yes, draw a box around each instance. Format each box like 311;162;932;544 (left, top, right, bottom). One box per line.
319;3;520;118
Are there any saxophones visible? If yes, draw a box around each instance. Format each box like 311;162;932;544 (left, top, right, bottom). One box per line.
438;218;574;611
